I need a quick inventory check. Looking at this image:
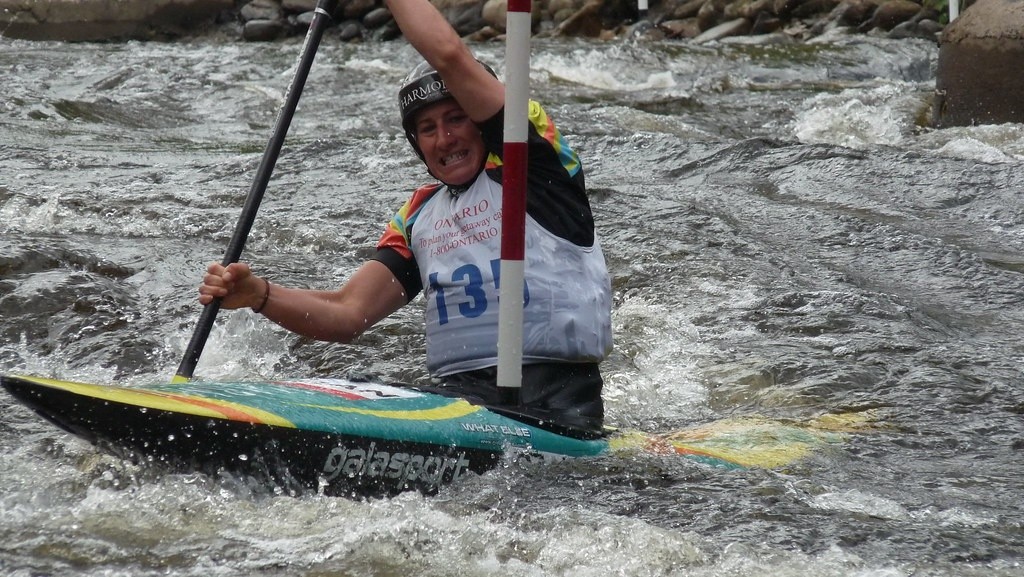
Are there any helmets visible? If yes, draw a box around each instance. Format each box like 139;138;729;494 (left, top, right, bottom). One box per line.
397;55;498;133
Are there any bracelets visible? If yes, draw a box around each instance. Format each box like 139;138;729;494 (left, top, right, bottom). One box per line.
252;278;270;313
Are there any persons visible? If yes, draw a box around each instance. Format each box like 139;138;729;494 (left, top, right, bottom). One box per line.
199;0;613;420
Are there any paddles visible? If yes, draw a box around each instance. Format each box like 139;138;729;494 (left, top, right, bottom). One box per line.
173;0;335;386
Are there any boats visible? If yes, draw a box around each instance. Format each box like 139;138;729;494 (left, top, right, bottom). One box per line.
2;372;882;501
